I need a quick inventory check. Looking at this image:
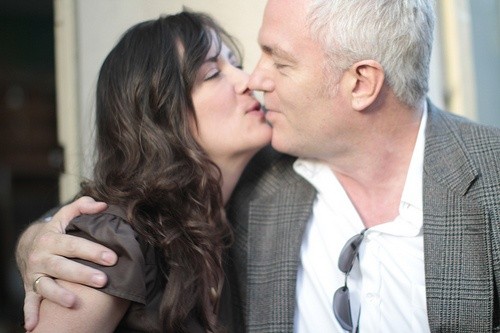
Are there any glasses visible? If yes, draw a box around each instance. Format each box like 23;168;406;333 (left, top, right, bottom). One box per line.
332;228;372;332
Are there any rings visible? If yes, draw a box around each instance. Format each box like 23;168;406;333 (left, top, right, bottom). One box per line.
33;275;50;295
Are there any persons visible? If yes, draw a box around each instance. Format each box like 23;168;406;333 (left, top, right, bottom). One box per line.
15;1;500;332
22;10;274;333
